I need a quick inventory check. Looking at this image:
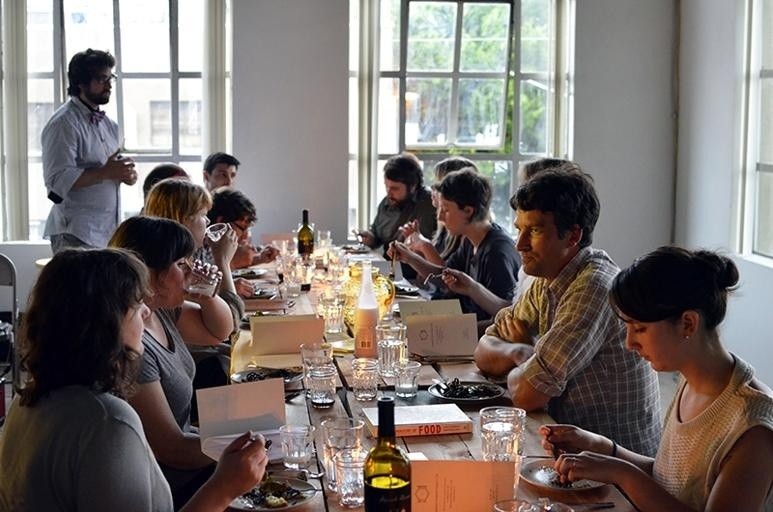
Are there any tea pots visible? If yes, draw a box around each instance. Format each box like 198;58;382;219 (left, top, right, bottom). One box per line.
340;265;395;326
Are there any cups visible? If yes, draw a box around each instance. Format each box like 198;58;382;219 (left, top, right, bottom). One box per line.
479;405;527;488
491;497;535;512
204;222;228;243
374;338;411;379
351;357;380;403
271;239;286;257
298;341;333;398
319;238;333;246
317;229;332;243
324;290;347;335
182;262;222;298
306;363;336;411
328;256;350;292
319;416;366;492
273;255;293;283
298;264;316;292
278;424;316;471
332;448;369;509
391;359;422;399
372;323;407;340
287;282;300;298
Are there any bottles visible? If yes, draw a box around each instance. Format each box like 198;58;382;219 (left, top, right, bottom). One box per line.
354;258;379;359
297;209;314;255
363;398;412;512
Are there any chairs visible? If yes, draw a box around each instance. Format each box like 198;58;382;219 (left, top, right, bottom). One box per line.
0;253;20;395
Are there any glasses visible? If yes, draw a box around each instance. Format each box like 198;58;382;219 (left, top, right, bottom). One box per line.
231;220;246;232
94;72;117;84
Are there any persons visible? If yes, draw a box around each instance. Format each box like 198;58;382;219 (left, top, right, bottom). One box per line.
399;156;496;265
441;159;580;318
473;162;663;460
41;47;138;254
538;247;772;511
0;248;268;512
107;217;234;490
387;167;522;319
208;187;280;271
144;177;245;334
203;153;241;193
351;151;437;262
144;162;190;206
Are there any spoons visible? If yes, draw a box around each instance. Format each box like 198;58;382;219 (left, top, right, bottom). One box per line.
424;273;447;284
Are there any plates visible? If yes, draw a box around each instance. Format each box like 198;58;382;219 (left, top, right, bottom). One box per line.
518;458;607;496
395;283;420;296
246;290;277;299
232;267;268;279
230;364;306;385
230;475;317;512
427;380;507;408
348;245;371;253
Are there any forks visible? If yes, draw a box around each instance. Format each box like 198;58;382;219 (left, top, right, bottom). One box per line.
389;240;396;281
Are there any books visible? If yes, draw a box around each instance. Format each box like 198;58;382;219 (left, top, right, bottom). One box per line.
361;402;473;439
